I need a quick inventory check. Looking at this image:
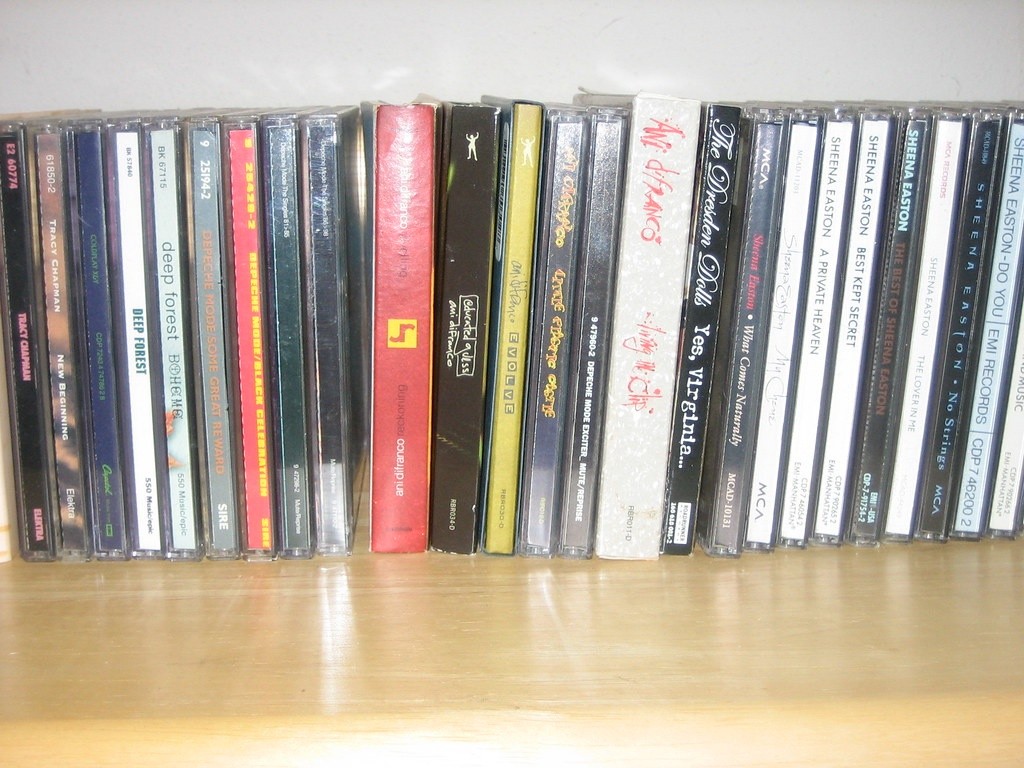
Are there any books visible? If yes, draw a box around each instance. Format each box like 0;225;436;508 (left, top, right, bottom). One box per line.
0;94;1024;562
